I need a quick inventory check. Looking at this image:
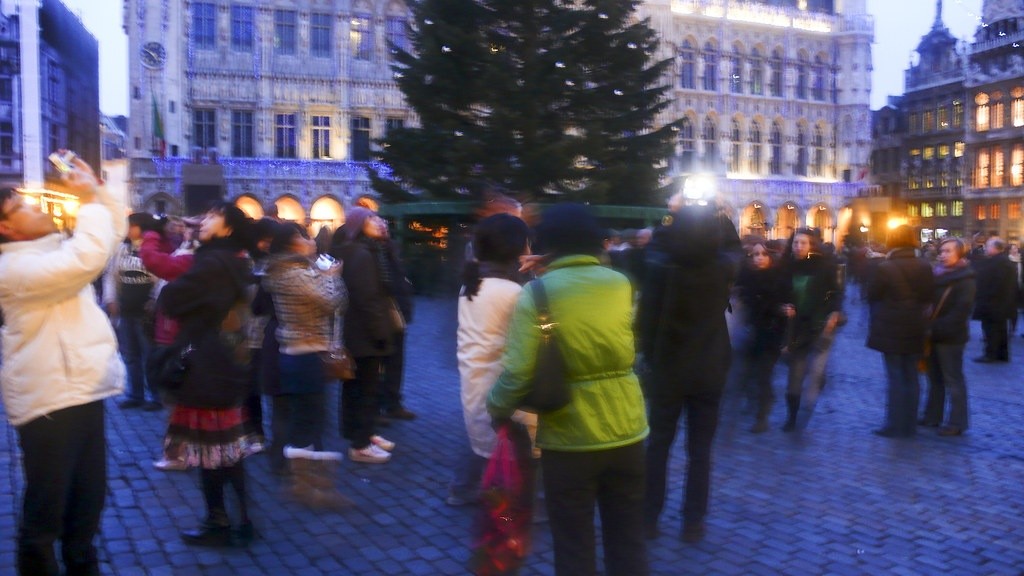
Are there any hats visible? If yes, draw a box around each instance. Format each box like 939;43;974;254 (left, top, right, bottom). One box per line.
342;207;373;240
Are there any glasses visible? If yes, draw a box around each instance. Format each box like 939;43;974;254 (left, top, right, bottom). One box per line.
4;196;36;221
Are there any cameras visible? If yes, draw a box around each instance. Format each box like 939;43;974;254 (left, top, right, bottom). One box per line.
49;150;76;173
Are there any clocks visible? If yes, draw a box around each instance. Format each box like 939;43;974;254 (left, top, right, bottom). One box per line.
137;41;166;71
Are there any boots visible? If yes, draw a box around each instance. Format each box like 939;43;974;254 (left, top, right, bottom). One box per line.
283;446;357;509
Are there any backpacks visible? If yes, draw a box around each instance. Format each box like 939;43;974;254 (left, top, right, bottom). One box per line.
203;252;257;364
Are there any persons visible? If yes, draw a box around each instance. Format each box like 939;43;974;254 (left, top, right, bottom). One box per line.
607;222;1023;364
917;237;971;435
0;149;129;576
774;230;846;434
152;204;264;549
486;202;656;576
729;240;784;429
635;210;734;543
94;209;415;426
455;215;541;576
861;226;939;436
264;221;356;512
334;207;402;465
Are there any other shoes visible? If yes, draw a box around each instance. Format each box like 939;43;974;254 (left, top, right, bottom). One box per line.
250;440;263;455
179;521;233;549
349;445;391;462
371;434;396;451
118;396;146;408
375;416;392;427
735;392;968;436
153;459;189;471
219;519;259;550
386;405;417;421
143;399;168;411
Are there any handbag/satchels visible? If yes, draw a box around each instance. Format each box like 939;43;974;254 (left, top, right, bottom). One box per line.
385;297;407;332
515;280;572;414
321;347;354;381
474;423;532;576
145;344;198;391
